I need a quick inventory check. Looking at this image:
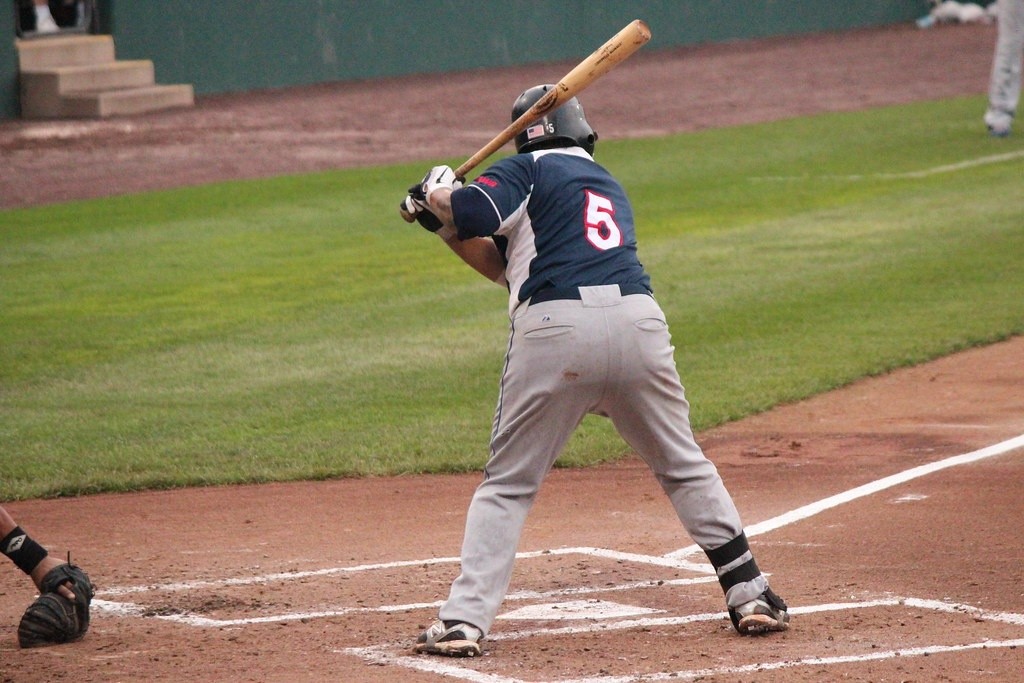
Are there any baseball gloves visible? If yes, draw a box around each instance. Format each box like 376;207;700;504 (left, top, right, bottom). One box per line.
15;548;95;651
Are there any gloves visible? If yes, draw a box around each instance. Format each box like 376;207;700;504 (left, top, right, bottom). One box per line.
402;165;464;232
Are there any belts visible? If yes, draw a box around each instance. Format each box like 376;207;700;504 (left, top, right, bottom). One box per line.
530;284;653;304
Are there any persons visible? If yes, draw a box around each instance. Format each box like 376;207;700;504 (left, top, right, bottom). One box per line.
0;505;93;602
400;83;788;654
984;0;1024;136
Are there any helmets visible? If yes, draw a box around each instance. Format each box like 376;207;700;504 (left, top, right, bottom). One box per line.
512;83;597;152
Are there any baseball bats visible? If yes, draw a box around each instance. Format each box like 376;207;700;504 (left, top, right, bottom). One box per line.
400;19;653;225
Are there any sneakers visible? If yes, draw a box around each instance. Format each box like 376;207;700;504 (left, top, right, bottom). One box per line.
726;589;791;635
413;620;481;658
984;107;1012;132
